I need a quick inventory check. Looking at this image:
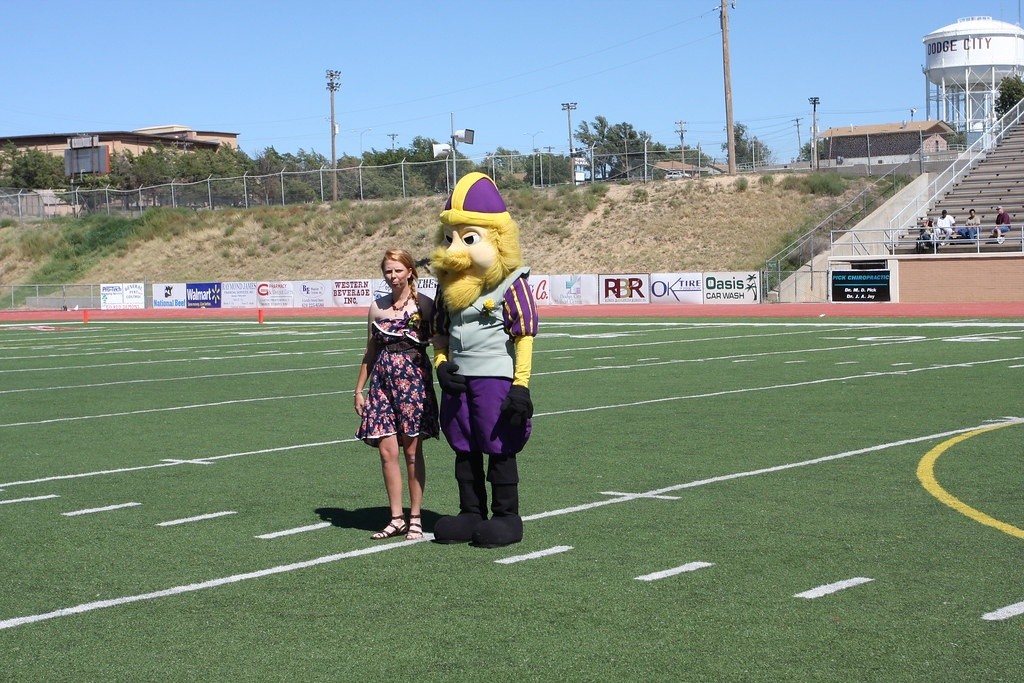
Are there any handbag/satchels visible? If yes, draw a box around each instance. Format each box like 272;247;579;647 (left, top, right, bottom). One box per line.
958;229;965;234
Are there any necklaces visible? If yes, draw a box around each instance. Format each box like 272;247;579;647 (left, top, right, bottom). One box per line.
391;292;412;311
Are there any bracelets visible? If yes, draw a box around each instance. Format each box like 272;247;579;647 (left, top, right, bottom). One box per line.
353;390;363;396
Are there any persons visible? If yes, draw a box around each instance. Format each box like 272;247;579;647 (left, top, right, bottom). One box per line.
993;205;1011;244
919;210;956;248
353;248;450;541
958;209;982;243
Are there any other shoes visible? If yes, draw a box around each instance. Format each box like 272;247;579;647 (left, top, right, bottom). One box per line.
939;242;945;245
998;240;1004;244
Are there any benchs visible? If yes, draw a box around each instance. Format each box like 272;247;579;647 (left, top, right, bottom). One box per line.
886;121;1024;254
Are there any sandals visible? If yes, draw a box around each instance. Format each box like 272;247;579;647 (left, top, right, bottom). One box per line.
406;514;423;540
371;514;408;539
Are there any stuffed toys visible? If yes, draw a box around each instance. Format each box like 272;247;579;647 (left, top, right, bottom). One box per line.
429;171;539;548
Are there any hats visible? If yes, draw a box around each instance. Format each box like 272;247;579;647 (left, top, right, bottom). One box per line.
942;210;947;213
996;206;1003;210
